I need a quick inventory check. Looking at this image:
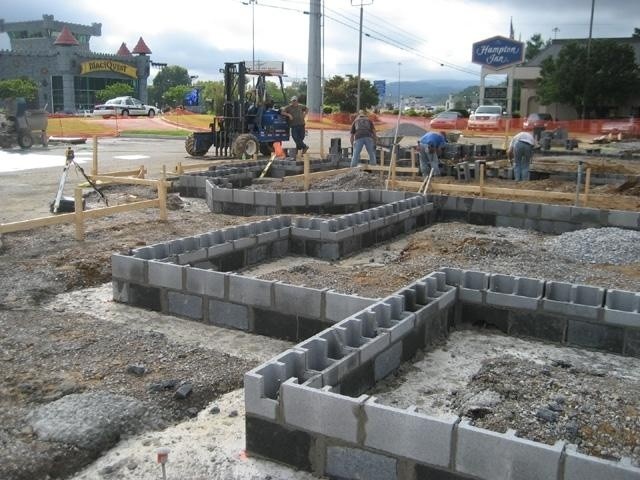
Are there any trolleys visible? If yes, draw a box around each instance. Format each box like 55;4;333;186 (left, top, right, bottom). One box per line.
446;130;463;143
373;134;405;151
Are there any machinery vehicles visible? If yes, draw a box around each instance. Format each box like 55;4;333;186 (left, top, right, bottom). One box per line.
0;97;50;148
184;58;296;160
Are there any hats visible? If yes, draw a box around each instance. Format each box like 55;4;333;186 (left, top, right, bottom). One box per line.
290;95;297;102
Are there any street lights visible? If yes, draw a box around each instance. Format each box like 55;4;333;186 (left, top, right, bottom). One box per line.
395;61;403;111
241;1;256;93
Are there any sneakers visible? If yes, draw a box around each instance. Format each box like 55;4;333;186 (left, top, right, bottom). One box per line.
302;145;309;153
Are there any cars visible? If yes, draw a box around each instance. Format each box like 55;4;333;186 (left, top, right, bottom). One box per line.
428;104;555;130
91;95;160;119
599;114;640;137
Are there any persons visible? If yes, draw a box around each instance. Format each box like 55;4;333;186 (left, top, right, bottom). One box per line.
506;132;537;181
415;130;446;178
280;95;310;154
350;112;378;167
